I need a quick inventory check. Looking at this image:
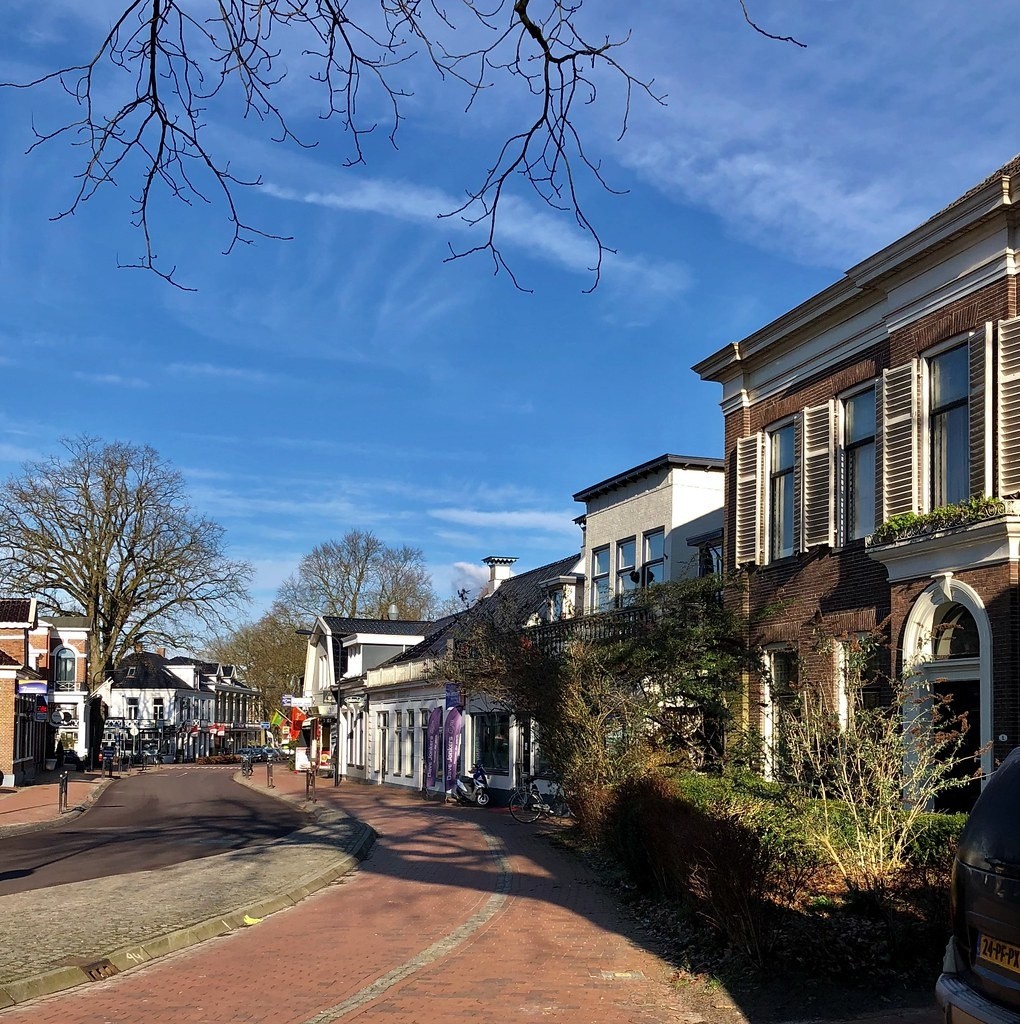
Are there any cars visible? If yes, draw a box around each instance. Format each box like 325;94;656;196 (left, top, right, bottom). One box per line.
253;748;268;762
237;748;255;763
262;748;285;761
934;745;1020;1024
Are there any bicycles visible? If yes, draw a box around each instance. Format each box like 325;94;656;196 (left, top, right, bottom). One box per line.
508;760;582;825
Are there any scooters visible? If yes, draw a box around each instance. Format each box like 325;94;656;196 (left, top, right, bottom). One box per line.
455;764;495;807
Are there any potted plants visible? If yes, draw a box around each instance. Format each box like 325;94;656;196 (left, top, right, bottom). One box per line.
46;736;58;770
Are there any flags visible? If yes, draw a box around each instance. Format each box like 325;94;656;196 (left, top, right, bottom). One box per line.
191;725;198;737
292;707;307;730
271;712;287;729
218;727;224;736
210;726;216;734
